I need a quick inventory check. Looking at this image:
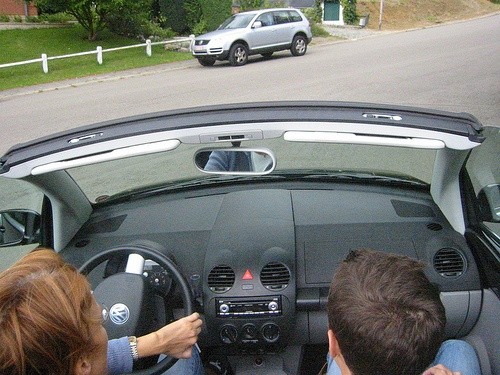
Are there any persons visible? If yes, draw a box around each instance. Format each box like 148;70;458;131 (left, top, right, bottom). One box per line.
326;248;482;375
204;150;274;172
0;249;203;375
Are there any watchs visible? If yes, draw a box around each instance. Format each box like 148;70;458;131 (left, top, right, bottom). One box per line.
128;336;138;362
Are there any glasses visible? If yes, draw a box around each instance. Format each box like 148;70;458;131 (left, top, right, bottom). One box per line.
86;303;110;342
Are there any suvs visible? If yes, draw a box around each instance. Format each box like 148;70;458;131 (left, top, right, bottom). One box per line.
189;8;314;67
